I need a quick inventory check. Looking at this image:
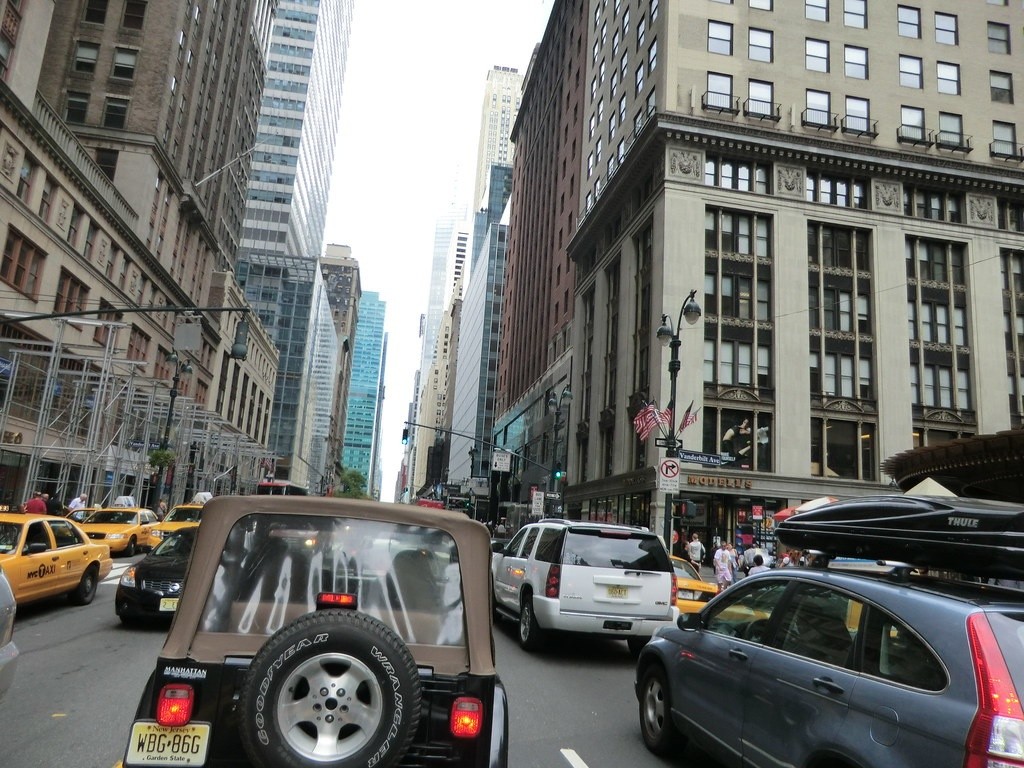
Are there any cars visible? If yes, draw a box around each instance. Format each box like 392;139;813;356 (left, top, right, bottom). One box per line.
115;525;199;630
669;554;900;639
258;518;514;611
147;492;214;555
0;508;112;649
72;495;160;558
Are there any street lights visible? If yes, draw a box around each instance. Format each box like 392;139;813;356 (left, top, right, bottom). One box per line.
656;289;701;555
546;384;573;518
151;350;194;512
468;444;479;519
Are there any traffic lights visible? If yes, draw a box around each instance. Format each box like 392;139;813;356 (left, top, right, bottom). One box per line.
553;460;562;480
402;429;408;445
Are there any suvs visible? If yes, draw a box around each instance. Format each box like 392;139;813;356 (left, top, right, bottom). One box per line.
638;494;1024;768
490;517;681;662
119;495;509;768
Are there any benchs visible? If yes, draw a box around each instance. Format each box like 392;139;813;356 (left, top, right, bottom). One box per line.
230;598;464;645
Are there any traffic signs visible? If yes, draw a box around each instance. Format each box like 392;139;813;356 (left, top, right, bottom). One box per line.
492;448;511;472
545;491;561;500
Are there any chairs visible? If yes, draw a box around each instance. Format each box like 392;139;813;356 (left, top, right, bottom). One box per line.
382;549;446;612
788;613;855;658
249;543;312;604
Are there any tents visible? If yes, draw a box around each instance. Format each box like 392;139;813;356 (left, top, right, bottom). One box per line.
904;477;958;497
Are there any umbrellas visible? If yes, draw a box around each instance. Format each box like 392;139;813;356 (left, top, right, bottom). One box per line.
772;497;839;521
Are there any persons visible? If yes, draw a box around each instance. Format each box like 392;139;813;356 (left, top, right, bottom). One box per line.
158;499;166;522
713;540;811;595
724;418;760;469
483;519;505;537
681;534;706;576
24;492;64;517
69;494;87;521
796;601;825;636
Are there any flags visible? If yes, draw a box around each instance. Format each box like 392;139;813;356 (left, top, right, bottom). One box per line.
679;401;698;433
633;399;671;441
